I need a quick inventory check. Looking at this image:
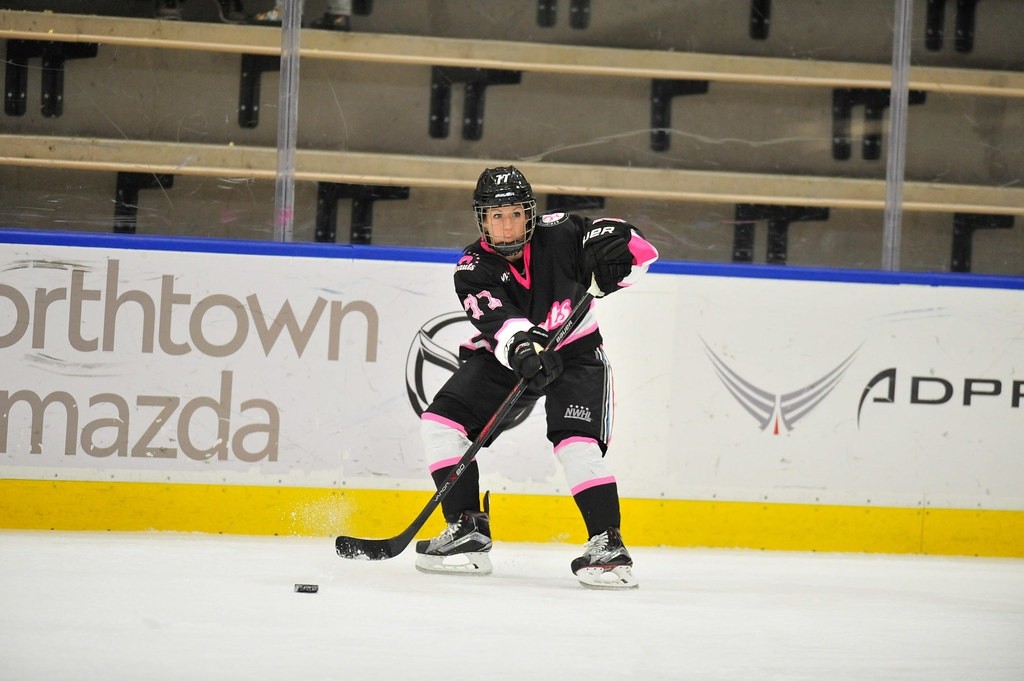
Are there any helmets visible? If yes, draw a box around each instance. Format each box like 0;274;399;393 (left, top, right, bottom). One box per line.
472;165;536;257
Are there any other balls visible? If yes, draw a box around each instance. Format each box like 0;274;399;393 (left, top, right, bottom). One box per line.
294;583;320;594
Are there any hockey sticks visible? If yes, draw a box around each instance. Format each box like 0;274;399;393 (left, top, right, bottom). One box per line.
333;292;592;561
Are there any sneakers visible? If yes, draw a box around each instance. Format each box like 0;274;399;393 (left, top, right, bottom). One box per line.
571;528;639;590
415;490;493;574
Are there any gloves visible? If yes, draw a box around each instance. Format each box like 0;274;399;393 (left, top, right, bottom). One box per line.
583;221;634;293
504;326;563;391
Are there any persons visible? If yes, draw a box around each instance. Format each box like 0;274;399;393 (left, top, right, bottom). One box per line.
414;166;659;590
